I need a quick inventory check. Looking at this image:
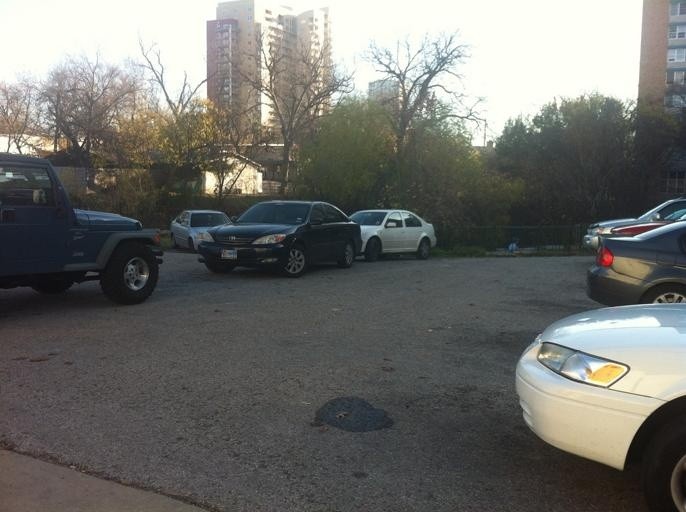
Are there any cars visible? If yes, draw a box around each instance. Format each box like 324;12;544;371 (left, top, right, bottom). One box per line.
169;209;232;252
516;303;685;511
348;209;438;260
583;196;685;307
198;202;362;277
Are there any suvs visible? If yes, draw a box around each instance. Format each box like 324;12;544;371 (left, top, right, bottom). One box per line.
0;152;162;302
